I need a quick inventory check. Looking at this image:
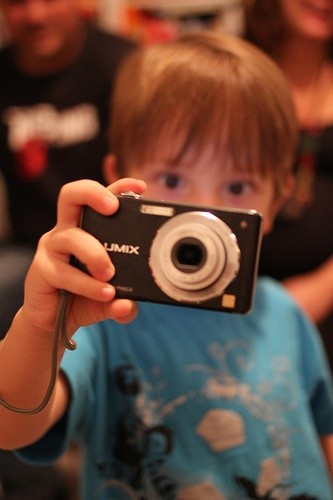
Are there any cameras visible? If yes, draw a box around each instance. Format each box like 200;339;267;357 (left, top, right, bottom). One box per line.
75;192;263;314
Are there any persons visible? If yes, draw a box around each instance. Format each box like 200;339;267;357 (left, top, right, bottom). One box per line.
234;0;333;369
0;0;140;341
0;31;333;500
